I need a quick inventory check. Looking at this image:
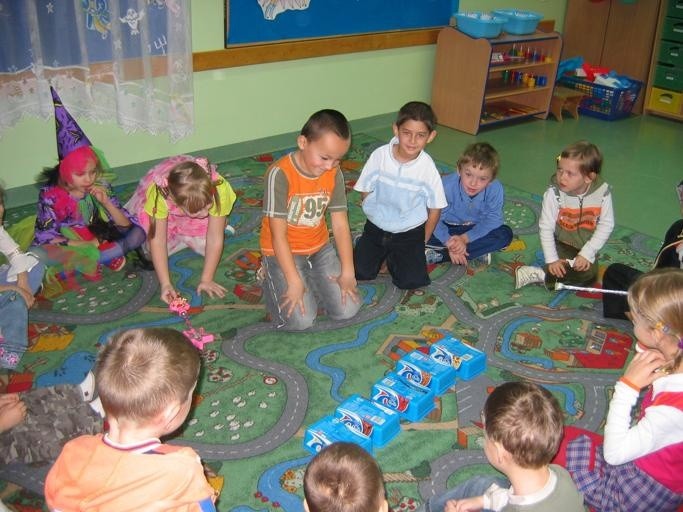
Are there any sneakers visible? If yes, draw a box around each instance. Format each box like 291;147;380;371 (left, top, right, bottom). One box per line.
475;253;491;266
92;359;102;401
109;257;125;272
516;264;544;290
424;247;442;264
224;225;235;237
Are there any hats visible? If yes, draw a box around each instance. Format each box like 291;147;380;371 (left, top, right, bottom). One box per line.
49;85;92;160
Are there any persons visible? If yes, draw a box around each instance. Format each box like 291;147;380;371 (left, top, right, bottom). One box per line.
27;84;140;282
302;440;390;512
0;189;47;369
0;371;118;476
353;101;447;290
512;140;615;289
602;178;683;319
44;326;219;512
415;380;583;511
254;109;364;331
425;142;513;269
552;269;683;512
124;154;236;302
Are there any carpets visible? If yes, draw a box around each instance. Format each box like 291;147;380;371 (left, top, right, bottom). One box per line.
0;131;683;507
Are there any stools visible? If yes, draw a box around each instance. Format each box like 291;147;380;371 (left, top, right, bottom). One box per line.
550;85;585;122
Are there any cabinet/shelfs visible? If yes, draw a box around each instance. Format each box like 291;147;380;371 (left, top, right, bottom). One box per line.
432;19;564;136
642;7;683;123
558;0;659;115
